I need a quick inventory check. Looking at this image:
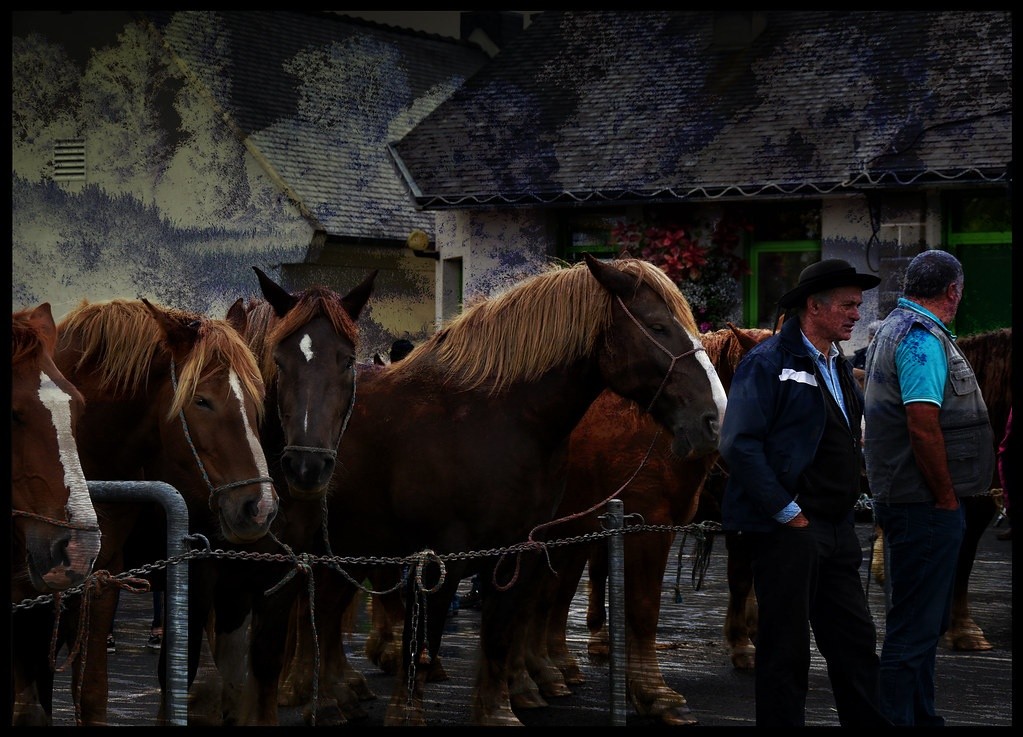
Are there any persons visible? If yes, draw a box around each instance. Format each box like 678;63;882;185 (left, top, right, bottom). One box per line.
851;319;885;388
721;260;882;727
864;250;994;727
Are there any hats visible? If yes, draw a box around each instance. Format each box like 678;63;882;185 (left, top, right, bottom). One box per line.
777;259;882;309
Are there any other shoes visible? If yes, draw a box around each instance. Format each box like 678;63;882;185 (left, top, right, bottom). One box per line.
914;713;945;727
997;527;1011;541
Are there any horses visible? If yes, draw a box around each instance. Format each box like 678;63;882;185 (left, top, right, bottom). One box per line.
13;255;1013;723
